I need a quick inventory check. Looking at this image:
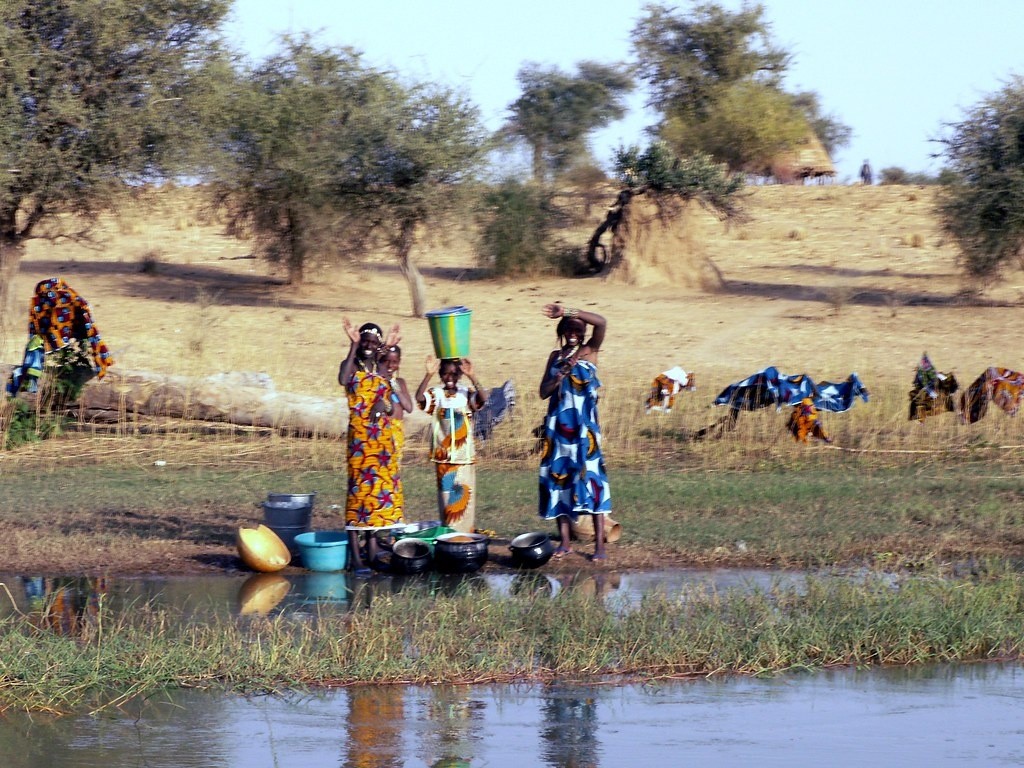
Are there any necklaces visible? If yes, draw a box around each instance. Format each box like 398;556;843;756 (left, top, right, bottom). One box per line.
357;355;379;374
558;344;582;360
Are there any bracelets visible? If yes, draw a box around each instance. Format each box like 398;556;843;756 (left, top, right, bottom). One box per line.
563;307;578;318
389;376;402;393
378;398;392;413
474;381;485;392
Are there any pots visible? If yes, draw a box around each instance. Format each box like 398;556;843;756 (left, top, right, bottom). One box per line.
392;537;434;573
435;531;489;572
509;531;554;569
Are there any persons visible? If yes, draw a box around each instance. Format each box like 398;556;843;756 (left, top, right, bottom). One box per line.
338;315;405;575
376;322;413;474
537;302;613;562
414;354;488;533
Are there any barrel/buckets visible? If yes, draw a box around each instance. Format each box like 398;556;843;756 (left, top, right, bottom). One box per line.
424;305;471;359
268;490;316;532
263;501;311;555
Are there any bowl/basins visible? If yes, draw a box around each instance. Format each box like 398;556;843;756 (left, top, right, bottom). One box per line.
394;519;443;534
294;531;350;571
236;521;291;572
396;526;453;552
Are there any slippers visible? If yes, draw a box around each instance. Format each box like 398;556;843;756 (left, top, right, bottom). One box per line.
366;556;390;571
377;546;395;556
554;545;574;557
356;545;367;558
592;554;609;562
352;565;371;578
